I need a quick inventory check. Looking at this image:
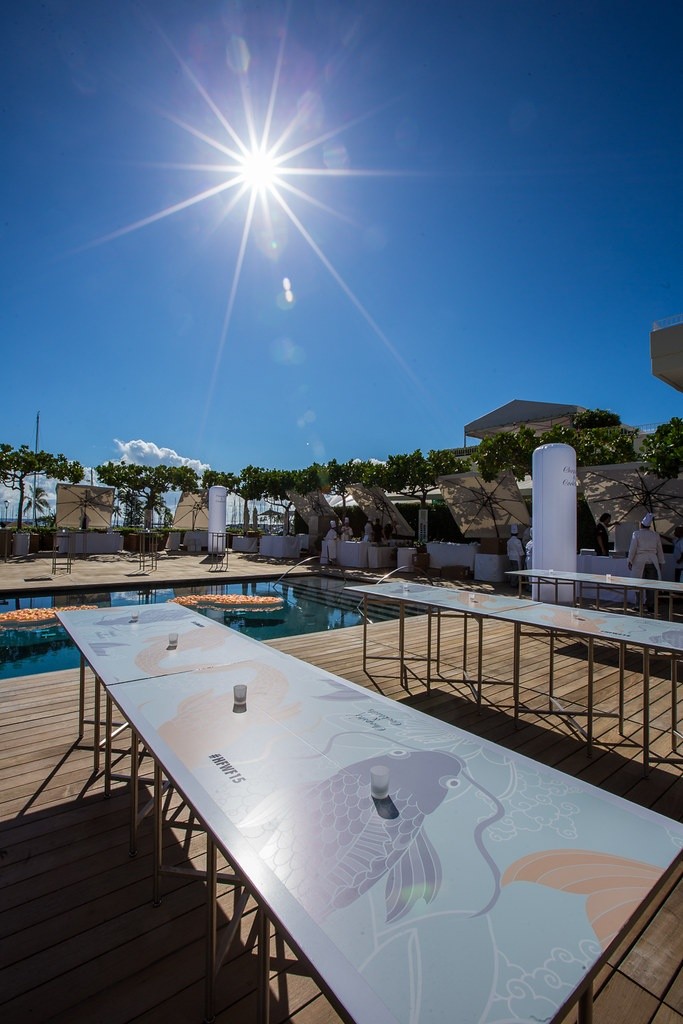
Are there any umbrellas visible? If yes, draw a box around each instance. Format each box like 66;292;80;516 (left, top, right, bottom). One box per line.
436;466;532;538
256;508;281;531
346;480;415;537
171;489;209;531
54;483;115;529
577;463;683;536
285;487;343;532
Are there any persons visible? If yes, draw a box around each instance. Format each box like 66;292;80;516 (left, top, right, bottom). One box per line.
506;523;533;591
658;527;683;582
592;513;620;557
326;521;338;567
364;517;393;542
627;513;666;613
340;517;353;542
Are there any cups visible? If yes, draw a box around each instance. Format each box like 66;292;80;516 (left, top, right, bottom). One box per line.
146;528;148;530
169;632;178;647
148;530;150;533
369;766;390;800
79;527;82;530
131;611;139;622
403;583;411;592
606;574;612;580
62;529;65;533
548;568;554;574
571;609;580;619
468;592;476;600
234;684;248;705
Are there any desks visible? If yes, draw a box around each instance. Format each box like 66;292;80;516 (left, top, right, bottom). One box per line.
140;532;158;570
425;543;480;574
582;575;683;660
262;536;300;558
232;537;258;554
55;602;287;863
183;531;208;551
368;547;397;568
55;532;120;554
322;540;371;568
106;657;683;1024
52;535;72;574
489;602;683;784
209;531;231;571
0;527;13;563
345;582;545;716
505;569;599;640
474;554;509;584
577;555;645;604
